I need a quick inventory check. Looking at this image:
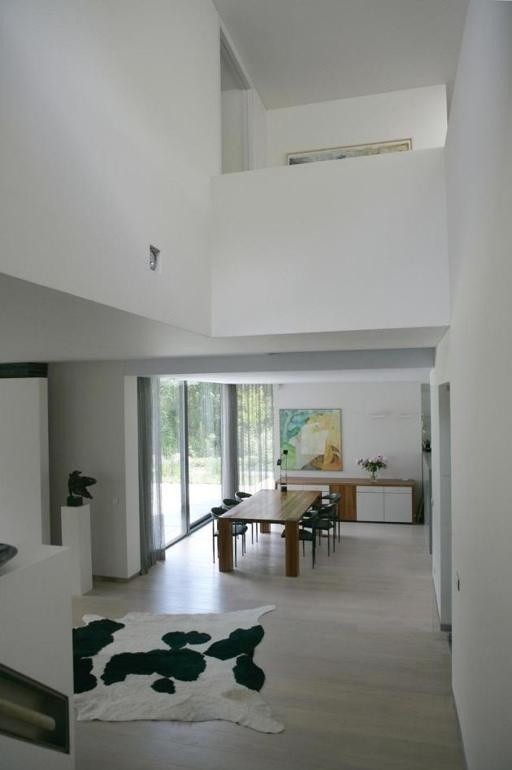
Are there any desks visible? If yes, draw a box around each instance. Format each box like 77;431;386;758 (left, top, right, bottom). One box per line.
217;489;322;577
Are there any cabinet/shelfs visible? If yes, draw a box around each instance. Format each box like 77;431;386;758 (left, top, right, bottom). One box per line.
275;477;419;525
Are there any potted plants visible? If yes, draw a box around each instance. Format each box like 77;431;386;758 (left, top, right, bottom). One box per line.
67;469;97;507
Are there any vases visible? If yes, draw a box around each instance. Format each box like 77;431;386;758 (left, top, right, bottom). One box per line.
369;472;377;482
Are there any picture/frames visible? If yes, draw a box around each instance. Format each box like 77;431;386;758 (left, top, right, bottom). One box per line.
285;137;414;167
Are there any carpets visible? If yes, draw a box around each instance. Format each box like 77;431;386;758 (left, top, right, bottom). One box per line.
72;604;286;736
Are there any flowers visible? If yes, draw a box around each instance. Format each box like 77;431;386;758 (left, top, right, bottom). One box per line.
354;452;392;482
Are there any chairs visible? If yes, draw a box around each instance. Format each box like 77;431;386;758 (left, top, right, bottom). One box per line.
211;491;342;570
